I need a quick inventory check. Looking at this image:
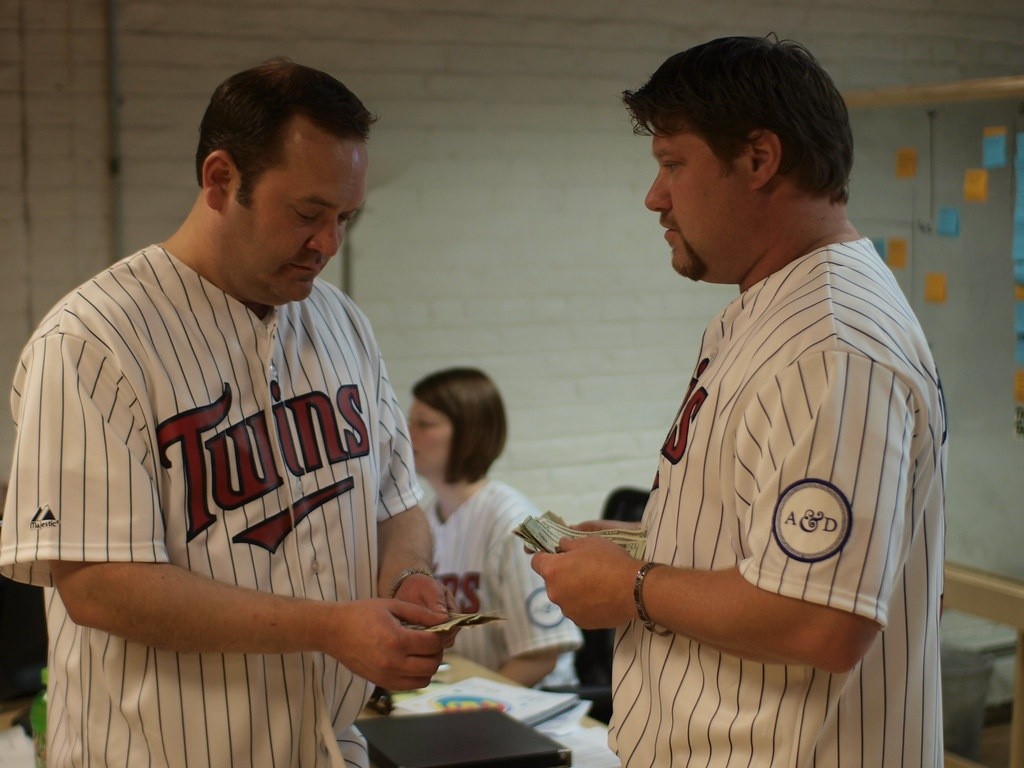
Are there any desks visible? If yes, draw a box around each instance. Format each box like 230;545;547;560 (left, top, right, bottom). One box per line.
358;650;608;729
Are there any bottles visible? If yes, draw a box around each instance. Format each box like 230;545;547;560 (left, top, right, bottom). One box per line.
30;668;50;768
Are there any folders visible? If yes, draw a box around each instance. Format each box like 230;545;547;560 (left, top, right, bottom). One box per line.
355;710;576;768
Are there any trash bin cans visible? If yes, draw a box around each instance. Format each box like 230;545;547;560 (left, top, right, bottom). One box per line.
938;646;993;758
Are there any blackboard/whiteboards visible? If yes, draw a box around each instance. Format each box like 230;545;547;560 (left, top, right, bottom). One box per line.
841;74;1024;637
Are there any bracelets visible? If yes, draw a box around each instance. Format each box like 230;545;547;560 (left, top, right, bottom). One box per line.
387;569;433;599
635;562;674;636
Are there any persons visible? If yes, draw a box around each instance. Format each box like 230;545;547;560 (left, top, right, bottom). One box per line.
531;37;946;768
407;368;589;703
1;60;462;768
0;575;49;768
